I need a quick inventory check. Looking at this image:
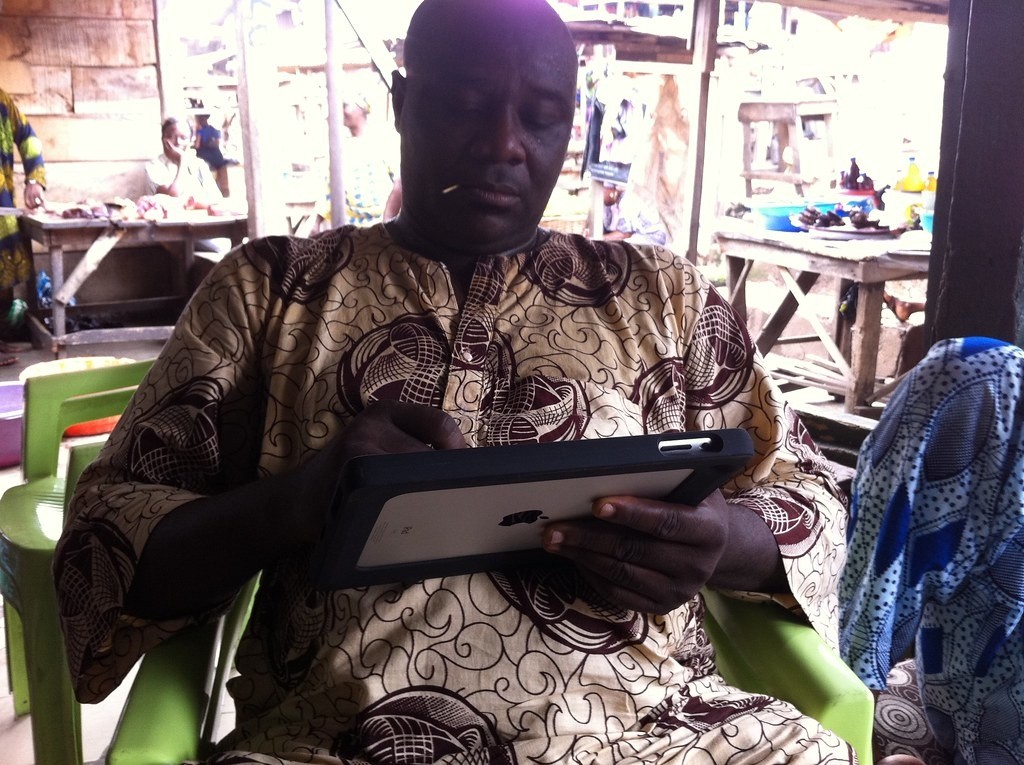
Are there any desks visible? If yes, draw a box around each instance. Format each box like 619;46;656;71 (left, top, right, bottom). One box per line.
716;223;932;414
21;196;249;365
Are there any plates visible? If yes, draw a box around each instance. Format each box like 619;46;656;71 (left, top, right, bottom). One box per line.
803;214;906;242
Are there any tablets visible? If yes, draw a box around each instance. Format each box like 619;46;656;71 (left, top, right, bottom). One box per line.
314;428;753;587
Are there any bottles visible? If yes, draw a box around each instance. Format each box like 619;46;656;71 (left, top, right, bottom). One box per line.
834;143;938;217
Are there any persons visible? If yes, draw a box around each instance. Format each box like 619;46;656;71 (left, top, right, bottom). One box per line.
0;83;48;368
48;0;856;765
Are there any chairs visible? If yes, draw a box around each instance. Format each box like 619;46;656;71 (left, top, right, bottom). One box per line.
0;355;874;765
